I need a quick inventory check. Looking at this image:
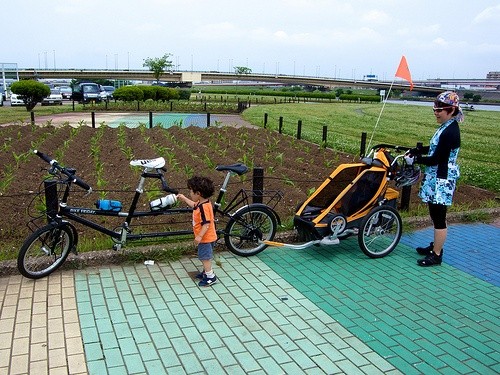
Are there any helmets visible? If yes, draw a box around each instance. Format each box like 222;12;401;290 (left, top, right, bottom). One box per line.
395;166;422;189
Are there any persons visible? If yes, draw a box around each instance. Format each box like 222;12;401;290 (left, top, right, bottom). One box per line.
176;176;217;287
404;91;461;267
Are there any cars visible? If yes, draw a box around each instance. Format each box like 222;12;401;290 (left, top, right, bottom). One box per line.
103;86;115;101
0;85;8;106
41;89;64;106
9;89;32;107
42;79;73;100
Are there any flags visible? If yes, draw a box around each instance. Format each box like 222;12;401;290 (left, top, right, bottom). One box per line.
394;56;414;92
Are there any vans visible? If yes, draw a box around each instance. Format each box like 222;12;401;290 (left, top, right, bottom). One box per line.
98;84;109;102
79;83;102;104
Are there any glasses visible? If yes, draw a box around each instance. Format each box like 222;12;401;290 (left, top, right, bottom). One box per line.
433;106;452;113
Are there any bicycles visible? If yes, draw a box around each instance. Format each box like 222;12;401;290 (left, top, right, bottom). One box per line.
17;149;283;280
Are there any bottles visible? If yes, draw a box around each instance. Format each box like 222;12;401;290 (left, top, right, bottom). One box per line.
94;199;122;211
149;194;180;211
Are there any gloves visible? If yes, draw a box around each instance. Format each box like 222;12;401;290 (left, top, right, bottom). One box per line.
410;145;428;164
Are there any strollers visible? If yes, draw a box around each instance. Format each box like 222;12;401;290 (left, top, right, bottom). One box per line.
259;140;432;257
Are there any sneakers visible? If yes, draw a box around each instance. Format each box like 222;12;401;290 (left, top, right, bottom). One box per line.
417;250;442;265
416;242;443;255
195;270;214;279
198;275;217;286
